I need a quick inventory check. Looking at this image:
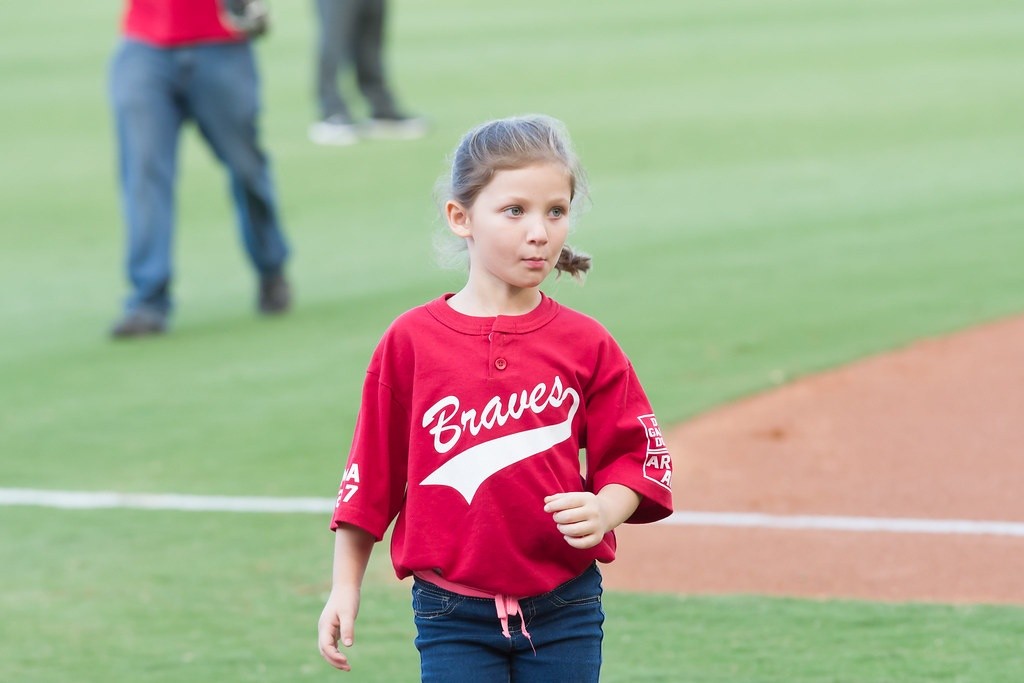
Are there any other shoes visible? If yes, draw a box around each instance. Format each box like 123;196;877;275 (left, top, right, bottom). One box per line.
366;115;426;141
314;120;358;145
260;270;286;313
109;312;165;337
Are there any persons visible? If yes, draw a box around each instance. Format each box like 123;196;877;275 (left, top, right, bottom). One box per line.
318;116;674;683
310;1;425;145
102;0;293;336
